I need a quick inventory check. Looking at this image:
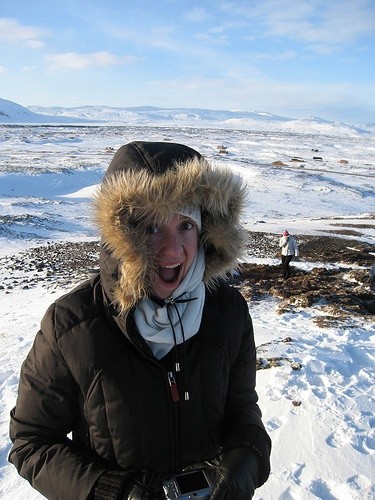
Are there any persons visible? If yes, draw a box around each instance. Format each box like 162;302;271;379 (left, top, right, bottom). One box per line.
7;141;270;500
279;229;299;279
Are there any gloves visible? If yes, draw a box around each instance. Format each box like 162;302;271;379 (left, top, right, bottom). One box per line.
180;445;260;500
118;466;167;500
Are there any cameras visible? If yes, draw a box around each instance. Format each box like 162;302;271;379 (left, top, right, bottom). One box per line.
162;468;214;500
